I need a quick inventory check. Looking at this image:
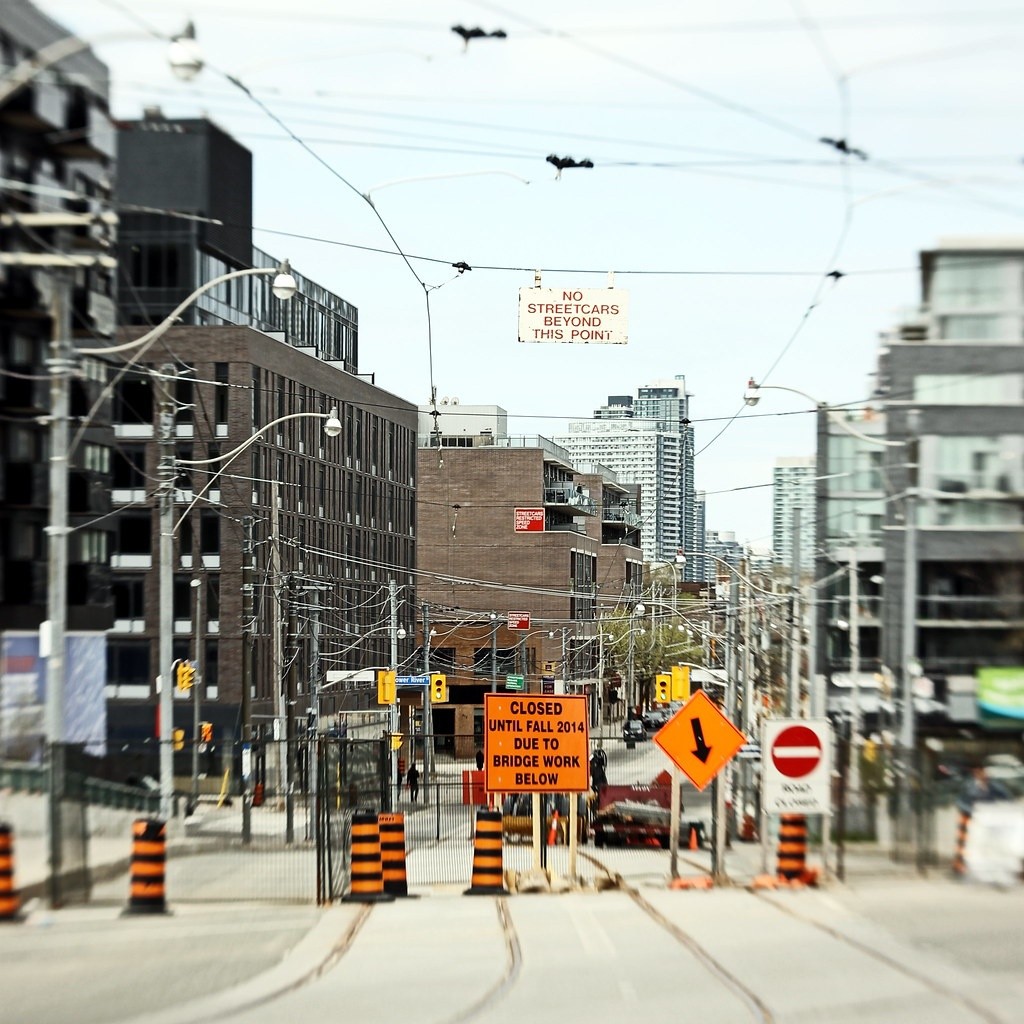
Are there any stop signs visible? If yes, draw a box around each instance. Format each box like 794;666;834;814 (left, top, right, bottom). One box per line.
770;726;825;778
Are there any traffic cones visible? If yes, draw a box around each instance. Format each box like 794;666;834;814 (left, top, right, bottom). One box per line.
689;828;698;849
548;810;559;846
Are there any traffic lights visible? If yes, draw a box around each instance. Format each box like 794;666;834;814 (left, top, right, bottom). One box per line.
201;722;212;742
177;662;196;691
388;731;404;751
431;675;446;704
656;673;671;704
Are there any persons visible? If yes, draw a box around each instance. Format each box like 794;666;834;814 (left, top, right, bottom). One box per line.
476;750;484;771
398;758;402;800
406;764;419;803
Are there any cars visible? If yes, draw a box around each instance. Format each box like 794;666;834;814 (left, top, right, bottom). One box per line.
622;681;719;742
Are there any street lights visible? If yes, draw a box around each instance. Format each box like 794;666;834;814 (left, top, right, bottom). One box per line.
598;625;646;740
423;605;495;777
45;241;294;806
308;582;405;840
633;548;800;715
561;627;614;695
491;611;553;694
745;377;924;838
157;399;341;821
